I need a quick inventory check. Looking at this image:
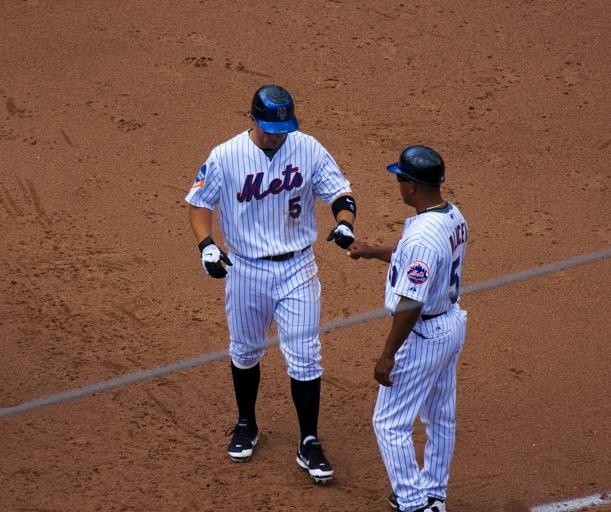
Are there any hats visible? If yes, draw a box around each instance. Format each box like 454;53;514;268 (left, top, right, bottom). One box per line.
250;83;299;135
387;144;447;187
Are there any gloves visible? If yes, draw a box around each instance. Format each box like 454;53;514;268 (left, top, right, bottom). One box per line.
197;234;233;280
326;219;355;250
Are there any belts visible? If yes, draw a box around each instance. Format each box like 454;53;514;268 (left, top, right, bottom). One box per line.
419;310;449;323
260;244;311;262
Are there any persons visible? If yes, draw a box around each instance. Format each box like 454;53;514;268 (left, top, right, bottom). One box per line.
346;146;469;512
184;85;355;484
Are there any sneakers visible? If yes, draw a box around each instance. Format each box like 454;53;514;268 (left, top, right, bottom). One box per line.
222;417;261;464
294;435;336;487
387;491;446;511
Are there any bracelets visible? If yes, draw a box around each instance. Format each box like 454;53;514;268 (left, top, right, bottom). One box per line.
332;195;356;225
